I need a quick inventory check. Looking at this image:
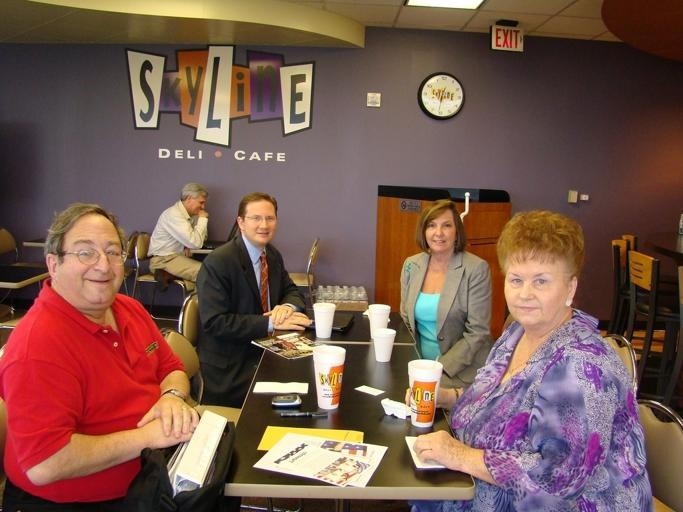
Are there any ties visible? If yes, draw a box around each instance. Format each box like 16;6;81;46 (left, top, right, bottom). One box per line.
259;251;268;314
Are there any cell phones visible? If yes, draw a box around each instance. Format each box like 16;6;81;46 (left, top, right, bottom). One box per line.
272;395;302;407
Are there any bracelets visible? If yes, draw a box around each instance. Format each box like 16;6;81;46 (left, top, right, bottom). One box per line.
160;388;187;402
451;386;459;403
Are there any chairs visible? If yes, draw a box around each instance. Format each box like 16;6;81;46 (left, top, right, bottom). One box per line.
286;234;321;306
0;228;47;303
118;231;240;428
605;234;682;511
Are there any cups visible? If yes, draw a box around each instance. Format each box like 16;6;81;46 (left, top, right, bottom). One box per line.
408;359;443;429
311;302;336;339
311;345;346;409
367;304;393;339
371;327;396;363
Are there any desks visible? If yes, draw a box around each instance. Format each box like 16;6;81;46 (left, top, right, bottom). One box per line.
0;265;48;291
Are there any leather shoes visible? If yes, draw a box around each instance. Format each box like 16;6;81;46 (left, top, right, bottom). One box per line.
154;268;170;293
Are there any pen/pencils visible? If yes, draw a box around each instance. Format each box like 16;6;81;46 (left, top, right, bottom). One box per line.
281;411;328;416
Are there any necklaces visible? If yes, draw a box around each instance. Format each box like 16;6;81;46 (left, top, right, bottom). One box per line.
508;342;529;375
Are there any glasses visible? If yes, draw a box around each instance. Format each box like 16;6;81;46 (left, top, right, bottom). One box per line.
243;215;276;224
57;248;129;267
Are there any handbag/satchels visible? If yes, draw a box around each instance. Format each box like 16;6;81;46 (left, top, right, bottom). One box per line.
120;422;236;510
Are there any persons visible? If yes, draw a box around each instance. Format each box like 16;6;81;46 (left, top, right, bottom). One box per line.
147;182;210;286
193;191;315;409
404;207;656;511
1;201;204;511
396;198;495;389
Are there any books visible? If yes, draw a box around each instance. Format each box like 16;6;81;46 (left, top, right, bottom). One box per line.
304;310;356;335
250;330;328;360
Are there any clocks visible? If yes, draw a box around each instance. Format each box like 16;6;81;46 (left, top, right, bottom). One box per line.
415;71;465;120
